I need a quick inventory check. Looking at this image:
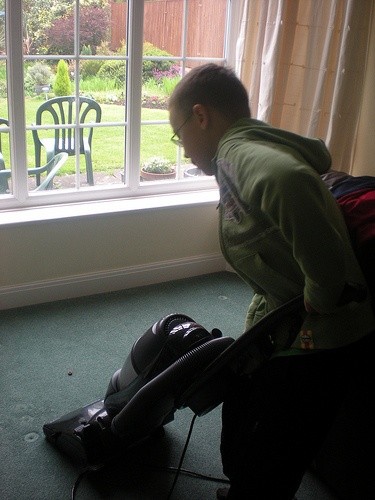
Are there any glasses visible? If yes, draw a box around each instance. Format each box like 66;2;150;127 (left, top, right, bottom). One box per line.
170;113;196;147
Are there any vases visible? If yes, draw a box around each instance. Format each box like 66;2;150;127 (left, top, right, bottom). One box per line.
184;166;213;177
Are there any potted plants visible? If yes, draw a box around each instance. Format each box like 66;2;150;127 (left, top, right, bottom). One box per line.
139;156;176;182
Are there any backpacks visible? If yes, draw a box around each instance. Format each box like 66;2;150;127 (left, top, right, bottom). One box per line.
318;170;375;284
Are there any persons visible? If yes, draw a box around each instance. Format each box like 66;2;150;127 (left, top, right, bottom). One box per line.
167;62;374;500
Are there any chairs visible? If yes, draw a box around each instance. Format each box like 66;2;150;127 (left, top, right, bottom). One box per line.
30;95;102;189
